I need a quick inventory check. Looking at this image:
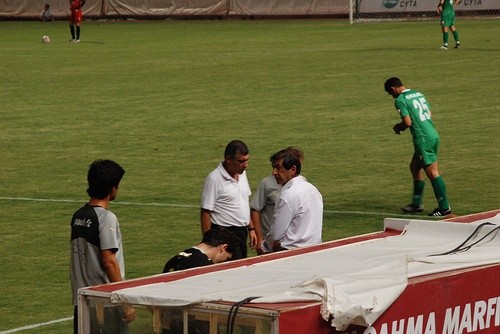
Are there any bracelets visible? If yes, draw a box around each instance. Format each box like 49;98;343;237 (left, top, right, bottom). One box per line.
248;228;256;231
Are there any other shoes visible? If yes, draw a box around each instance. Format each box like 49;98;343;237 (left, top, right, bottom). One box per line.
73;39;80;42
68;38;75;41
440;46;448;50
428;205;451;217
455;43;460;48
401;203;424;213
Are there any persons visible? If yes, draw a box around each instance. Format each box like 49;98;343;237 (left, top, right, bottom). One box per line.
200;140;257;261
162;228;244;334
251;147;303;255
40;4;54;22
261;150;324;255
384;77;453;217
69;0;87;42
70;159;136;334
436;0;461;49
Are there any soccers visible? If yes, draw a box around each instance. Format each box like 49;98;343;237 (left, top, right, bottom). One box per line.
42;36;50;43
